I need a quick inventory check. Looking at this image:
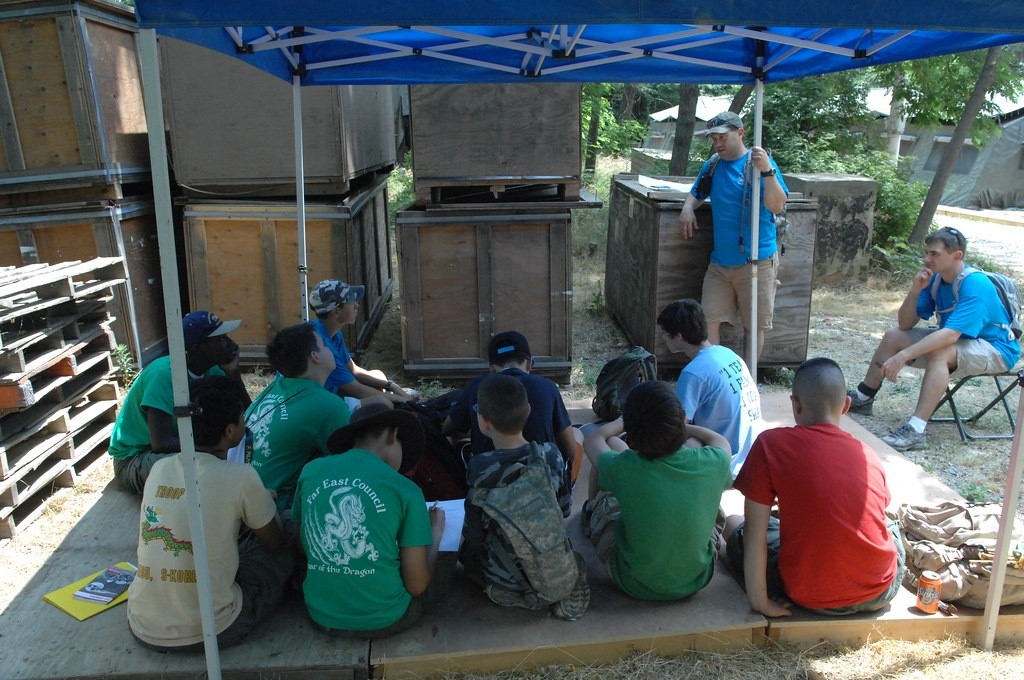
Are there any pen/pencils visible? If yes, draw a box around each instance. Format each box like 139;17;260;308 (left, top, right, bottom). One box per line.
430;500;439;512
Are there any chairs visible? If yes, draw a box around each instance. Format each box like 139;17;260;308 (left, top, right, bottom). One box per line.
926;364;1024;444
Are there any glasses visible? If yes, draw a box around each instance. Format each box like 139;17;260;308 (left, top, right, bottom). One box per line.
950;229;961;250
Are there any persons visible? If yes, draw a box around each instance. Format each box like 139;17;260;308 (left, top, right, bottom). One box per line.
108;310;253;497
680;111;789;375
127;375;286;653
290;403;445;640
656;298;761;484
448;374;591;620
275;278;412;410
722;358;908;617
442;330;585;487
583;381;732;601
243;321;350;516
847;226;1022;451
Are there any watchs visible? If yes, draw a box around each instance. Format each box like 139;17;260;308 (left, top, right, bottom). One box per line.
760;168;776;178
386;380;394;389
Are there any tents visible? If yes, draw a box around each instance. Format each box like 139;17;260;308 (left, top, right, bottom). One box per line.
132;0;1024;680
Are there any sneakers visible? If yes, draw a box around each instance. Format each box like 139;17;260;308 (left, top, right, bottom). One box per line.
846;389;873;415
883;424;926;452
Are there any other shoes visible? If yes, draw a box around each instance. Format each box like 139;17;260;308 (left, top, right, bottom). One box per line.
402;388;420;402
559;551;591;621
461;443;471;469
487;585;542;610
583;500;593;537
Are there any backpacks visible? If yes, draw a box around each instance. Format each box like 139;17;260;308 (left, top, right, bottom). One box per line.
983;272;1023;340
593;347;657;419
773;205;788;242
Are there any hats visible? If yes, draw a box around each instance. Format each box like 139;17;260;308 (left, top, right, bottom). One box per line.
705;111;743;138
488;331;530;362
309;279;365;314
183;311;242;350
326;403;425;474
